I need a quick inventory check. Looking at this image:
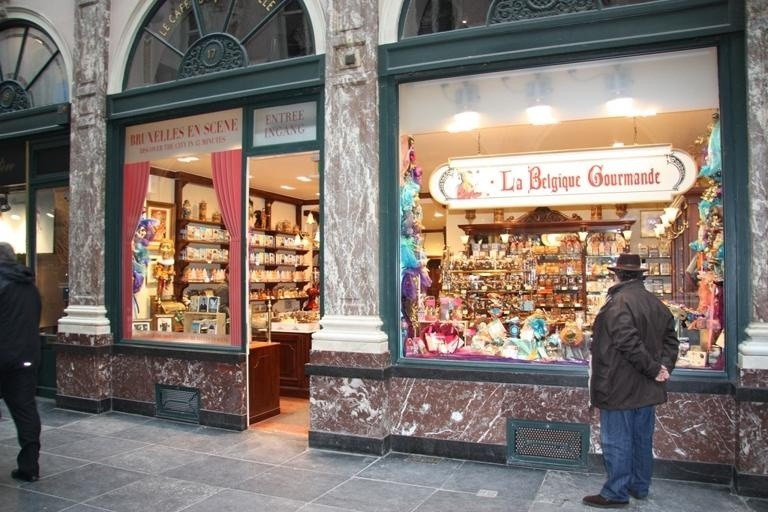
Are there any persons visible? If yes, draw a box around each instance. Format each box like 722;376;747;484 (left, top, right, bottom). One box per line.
1;241;42;484
582;252;680;510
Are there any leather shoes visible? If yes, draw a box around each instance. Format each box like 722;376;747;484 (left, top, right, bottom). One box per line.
12;469;38;481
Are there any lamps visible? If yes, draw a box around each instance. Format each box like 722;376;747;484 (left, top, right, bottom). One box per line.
446;83;485;132
604;76;634;117
525;81;555;126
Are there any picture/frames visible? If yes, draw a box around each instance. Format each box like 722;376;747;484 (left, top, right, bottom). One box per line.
158;317;171;334
145;201;177;252
132;322;151;334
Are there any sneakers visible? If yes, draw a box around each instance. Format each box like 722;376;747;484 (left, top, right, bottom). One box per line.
583;494;630;507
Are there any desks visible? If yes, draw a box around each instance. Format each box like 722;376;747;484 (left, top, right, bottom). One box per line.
413;320;469;347
250;342;281;424
252;322;315;399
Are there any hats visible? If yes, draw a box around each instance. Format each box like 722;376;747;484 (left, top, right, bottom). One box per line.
607;254;649;272
0;242;18;264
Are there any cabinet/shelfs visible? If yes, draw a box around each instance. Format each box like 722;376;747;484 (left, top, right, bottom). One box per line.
176;174;320;334
